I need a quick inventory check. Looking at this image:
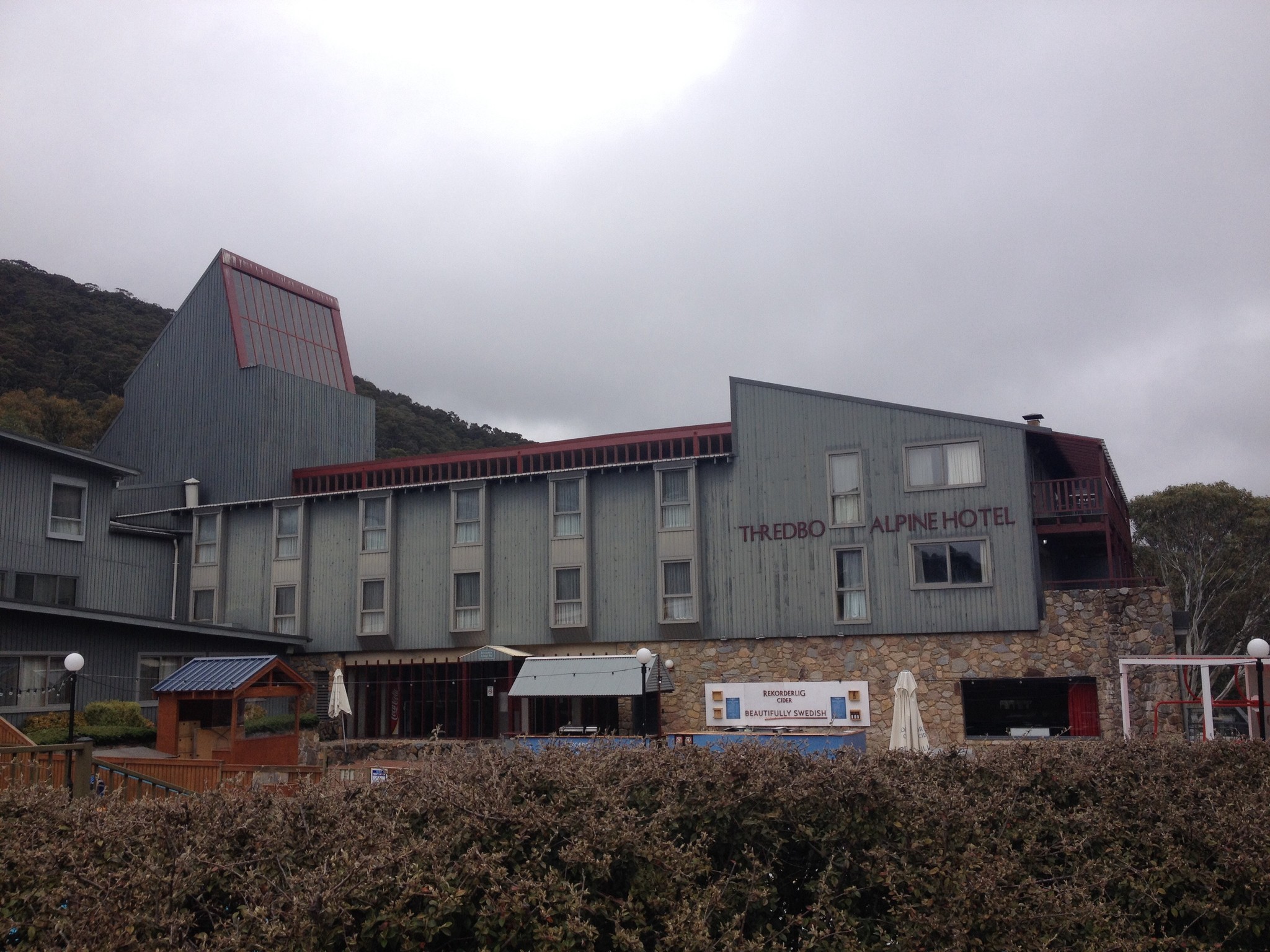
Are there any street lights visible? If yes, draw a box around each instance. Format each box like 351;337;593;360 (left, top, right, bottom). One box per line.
1246;638;1270;741
636;648;652;748
64;653;85;809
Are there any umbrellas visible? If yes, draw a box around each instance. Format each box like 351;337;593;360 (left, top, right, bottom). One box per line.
888;669;933;755
327;668;353;752
